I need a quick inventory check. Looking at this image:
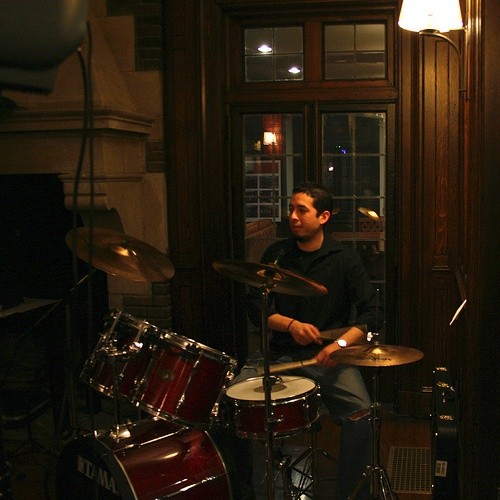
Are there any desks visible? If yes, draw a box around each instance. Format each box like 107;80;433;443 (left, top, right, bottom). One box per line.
333;232;383;250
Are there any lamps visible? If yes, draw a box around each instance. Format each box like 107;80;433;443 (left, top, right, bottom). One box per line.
396;0;468;91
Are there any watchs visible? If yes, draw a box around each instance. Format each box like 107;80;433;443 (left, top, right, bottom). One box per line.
334;339;348;349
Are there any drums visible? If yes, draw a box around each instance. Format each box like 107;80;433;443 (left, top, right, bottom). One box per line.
225;376;320;440
55;420;233;500
132;329;238;430
80;309;162;404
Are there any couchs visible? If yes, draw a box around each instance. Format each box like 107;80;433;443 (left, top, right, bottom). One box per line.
246;218;276;263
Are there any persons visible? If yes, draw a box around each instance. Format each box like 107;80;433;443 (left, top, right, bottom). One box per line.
228;178;385;500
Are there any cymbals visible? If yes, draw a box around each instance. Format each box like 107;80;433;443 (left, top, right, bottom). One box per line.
212;259;328;297
330;343;425;367
66;227;176;283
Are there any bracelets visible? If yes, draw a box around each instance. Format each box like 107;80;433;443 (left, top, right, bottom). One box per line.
286;319;295;333
